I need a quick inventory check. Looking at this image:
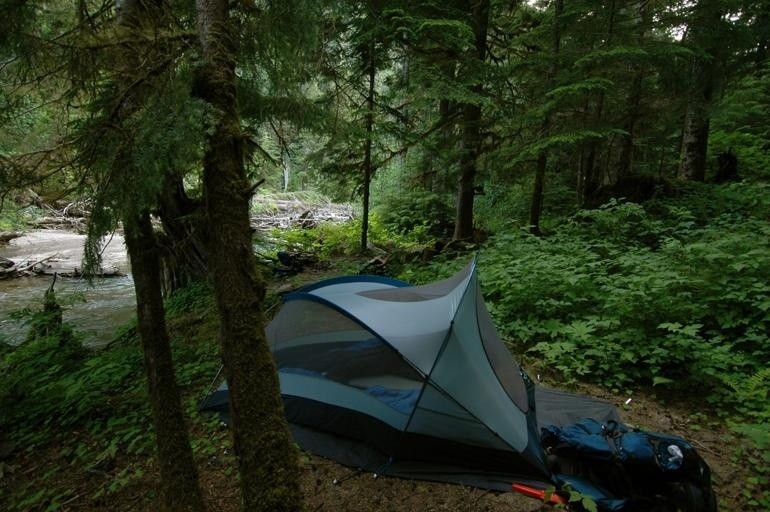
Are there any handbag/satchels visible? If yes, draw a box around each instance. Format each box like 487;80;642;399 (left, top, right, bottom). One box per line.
543;417;717;512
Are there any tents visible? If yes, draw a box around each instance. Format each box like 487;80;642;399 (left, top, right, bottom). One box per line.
197;251;554;492
